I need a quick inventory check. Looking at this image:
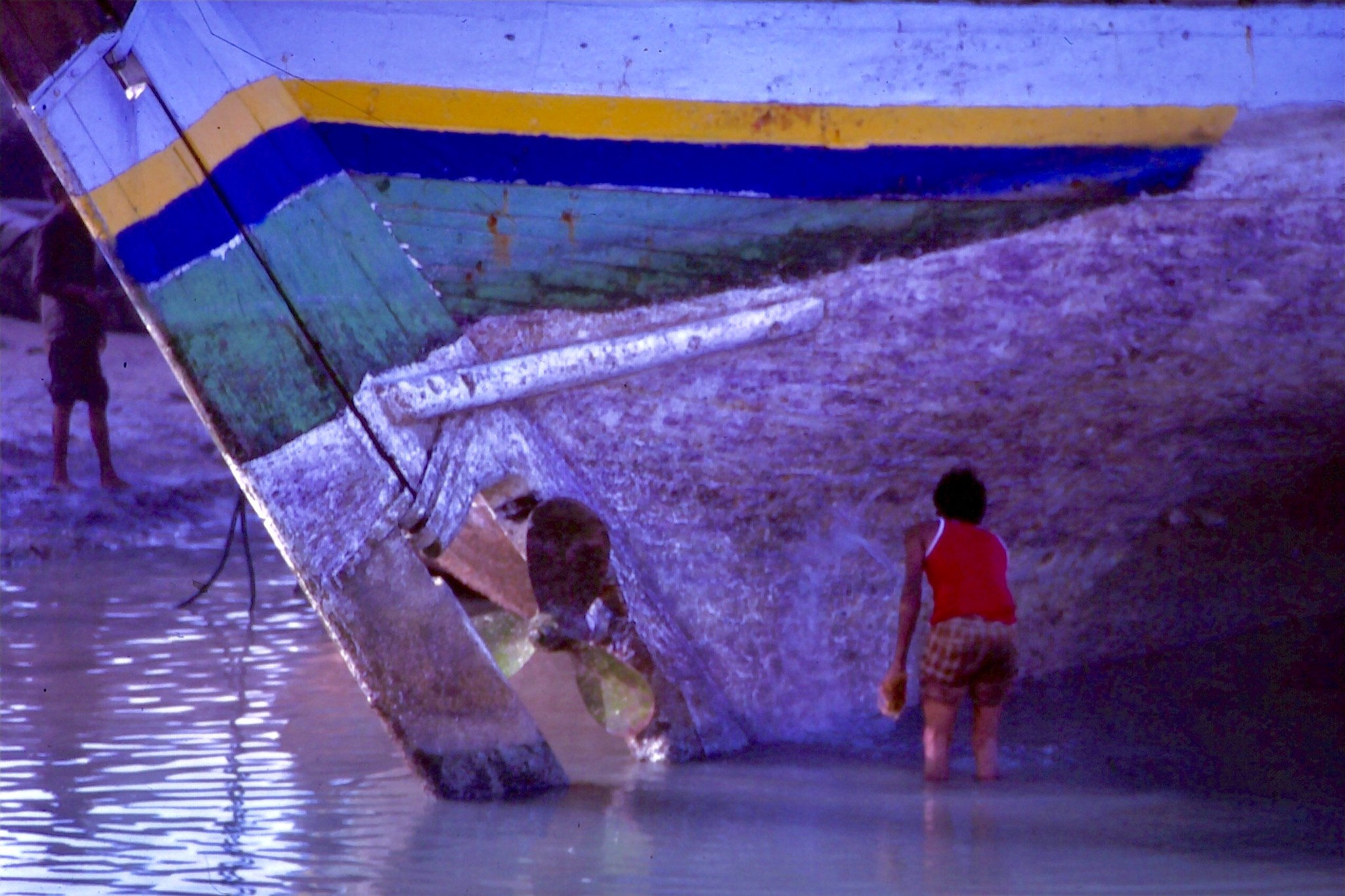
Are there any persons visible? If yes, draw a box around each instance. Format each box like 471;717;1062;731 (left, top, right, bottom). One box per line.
32;168;133;492
880;467;1020;788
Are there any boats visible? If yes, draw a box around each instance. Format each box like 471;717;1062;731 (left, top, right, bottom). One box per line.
3;2;1345;825
